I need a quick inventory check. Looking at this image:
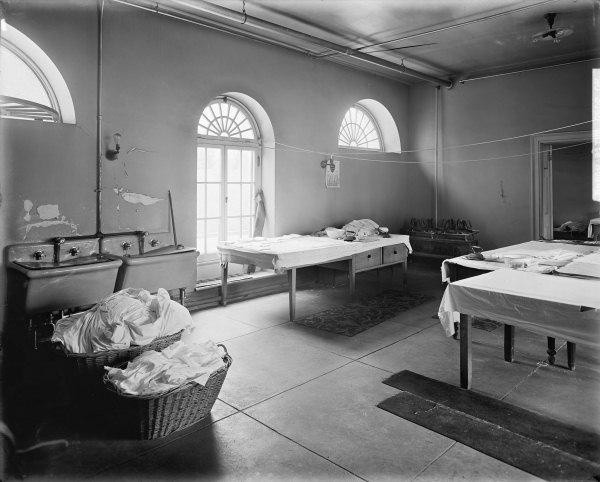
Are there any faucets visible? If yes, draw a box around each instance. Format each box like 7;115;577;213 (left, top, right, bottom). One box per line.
53;235;66;262
138;230;149;254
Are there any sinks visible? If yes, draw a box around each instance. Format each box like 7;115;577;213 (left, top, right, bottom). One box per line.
12;254;122;315
107;245;198;292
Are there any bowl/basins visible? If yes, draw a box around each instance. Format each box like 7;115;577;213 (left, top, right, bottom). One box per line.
325;227;346;239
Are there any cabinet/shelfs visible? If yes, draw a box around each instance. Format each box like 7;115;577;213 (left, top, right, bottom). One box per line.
309;234;413;299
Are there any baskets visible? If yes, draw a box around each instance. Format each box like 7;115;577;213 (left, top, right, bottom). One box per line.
48;311;233;440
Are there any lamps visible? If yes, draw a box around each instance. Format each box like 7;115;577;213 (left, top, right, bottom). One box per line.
531;13;573;46
106;132;122;160
321;153;335;173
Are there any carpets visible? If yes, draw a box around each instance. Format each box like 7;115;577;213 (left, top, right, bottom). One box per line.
433;314;502;332
376;370;600;482
293;289;430;337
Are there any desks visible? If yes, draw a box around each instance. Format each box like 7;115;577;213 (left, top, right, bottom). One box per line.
441;240;600;364
555;247;600;368
437;268;600;390
217;234;355;323
